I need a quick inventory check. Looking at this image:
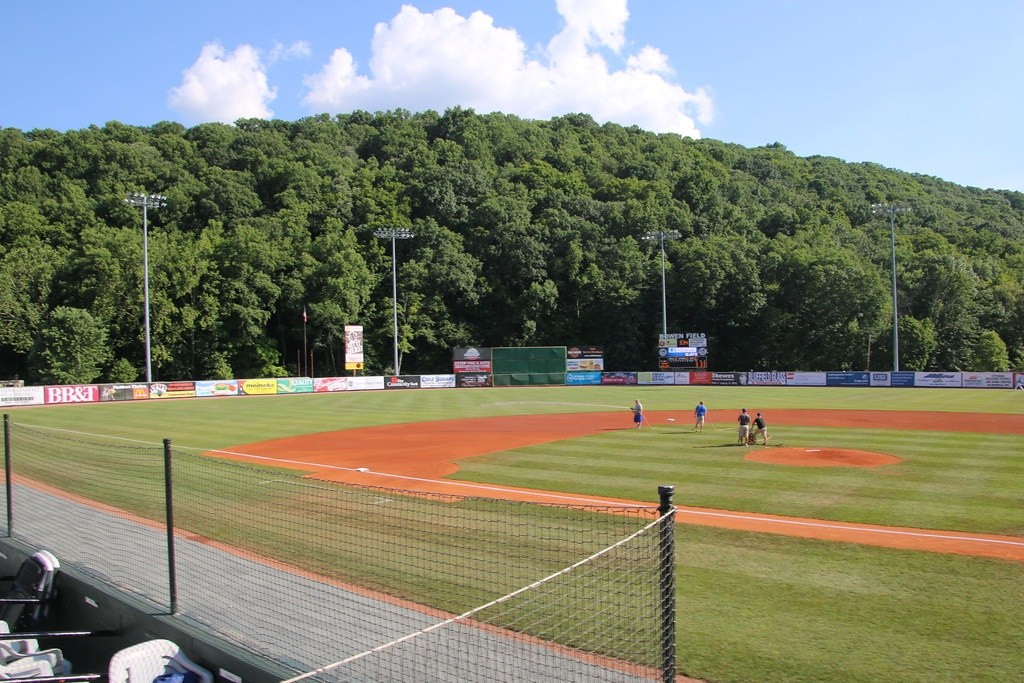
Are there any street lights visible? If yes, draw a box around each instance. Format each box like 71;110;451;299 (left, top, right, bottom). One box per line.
374;225;416;377
640;228;682;335
871;204;912;371
124;191;168;381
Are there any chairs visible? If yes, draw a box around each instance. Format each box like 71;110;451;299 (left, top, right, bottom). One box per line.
108;639;214;682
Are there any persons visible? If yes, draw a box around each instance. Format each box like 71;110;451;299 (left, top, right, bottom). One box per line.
751;412;768;446
694;400;707;434
633;398;643;431
736;407;751;446
